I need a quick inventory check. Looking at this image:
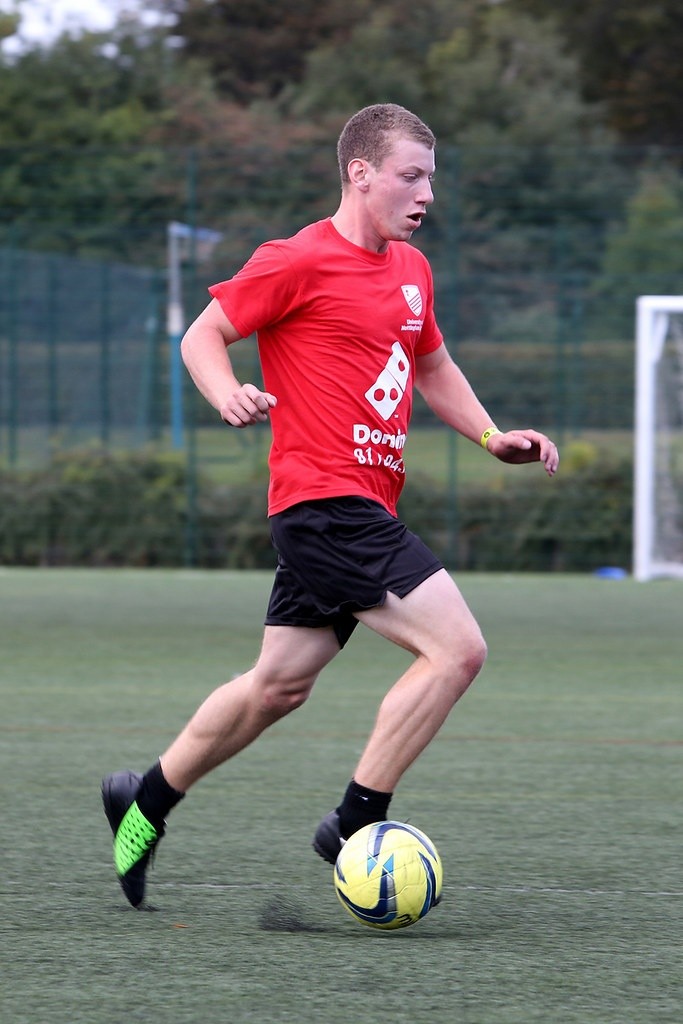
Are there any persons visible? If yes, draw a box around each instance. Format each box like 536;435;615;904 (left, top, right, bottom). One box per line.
99;107;559;912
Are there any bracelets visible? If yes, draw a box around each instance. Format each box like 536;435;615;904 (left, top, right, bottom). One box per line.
480;428;503;449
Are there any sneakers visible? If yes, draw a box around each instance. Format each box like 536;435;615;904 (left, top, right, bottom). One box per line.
312;809;443;907
102;769;167;908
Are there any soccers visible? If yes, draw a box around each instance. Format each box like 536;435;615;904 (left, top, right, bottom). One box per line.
334;821;443;929
